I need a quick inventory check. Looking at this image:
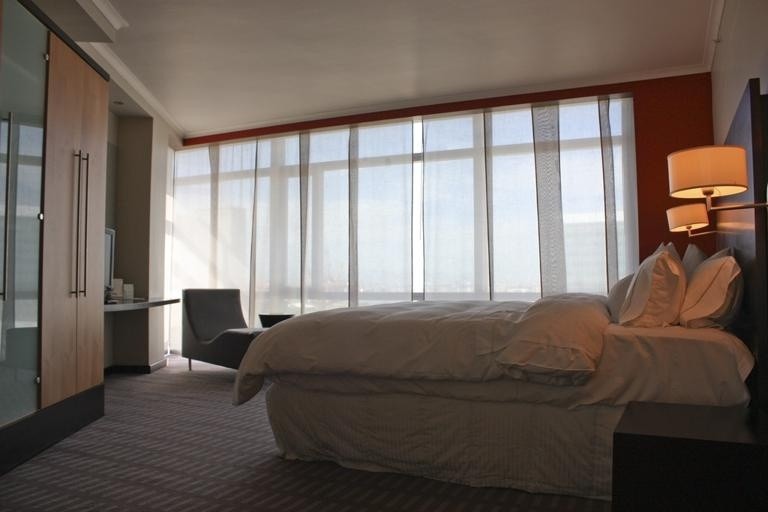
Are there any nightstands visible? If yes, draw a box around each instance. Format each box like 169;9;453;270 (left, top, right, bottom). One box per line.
612;400;768;512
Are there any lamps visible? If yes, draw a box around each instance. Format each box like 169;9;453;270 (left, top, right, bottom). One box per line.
667;146;768;211
665;202;716;238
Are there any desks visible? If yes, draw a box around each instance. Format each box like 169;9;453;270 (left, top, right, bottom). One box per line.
103;297;179;311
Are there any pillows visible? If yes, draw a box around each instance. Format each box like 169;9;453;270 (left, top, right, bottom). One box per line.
684;245;707;280
608;244;634;322
680;246;746;328
617;243;684;329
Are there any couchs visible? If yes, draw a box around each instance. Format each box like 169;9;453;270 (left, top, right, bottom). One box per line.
180;287;262;371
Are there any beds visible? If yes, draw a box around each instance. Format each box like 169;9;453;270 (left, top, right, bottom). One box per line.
262;300;753;499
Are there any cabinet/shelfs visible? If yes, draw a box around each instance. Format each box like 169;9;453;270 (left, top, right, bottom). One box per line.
38;32;109;408
1;1;43;427
